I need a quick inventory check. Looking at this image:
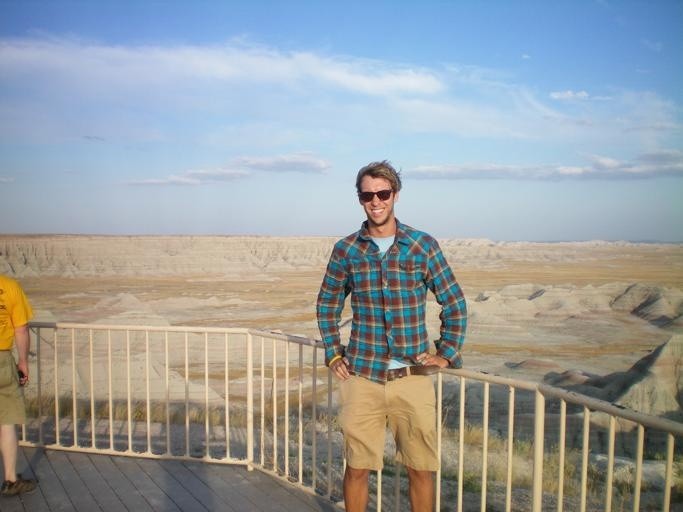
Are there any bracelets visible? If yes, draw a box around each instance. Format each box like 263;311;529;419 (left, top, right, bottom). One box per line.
327;355;342;367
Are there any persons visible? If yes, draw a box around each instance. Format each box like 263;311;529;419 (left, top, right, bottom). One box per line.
315;159;467;512
0;269;38;496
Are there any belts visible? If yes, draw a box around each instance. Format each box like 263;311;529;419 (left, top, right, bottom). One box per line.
388;365;441;381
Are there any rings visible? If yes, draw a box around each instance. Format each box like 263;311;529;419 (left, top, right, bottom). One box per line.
425;355;429;359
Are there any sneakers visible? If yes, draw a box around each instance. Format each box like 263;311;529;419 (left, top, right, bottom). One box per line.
0;474;38;496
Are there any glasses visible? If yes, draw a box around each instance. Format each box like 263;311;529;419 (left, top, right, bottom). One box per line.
359;190;393;202
